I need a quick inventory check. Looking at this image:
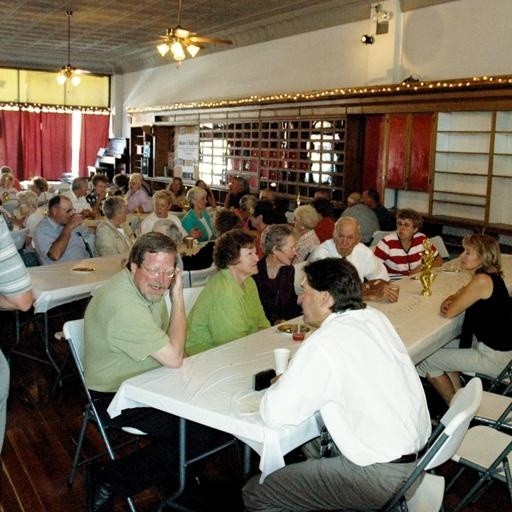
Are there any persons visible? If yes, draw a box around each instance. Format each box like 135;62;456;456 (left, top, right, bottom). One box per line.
0;209;37;480
282;188;397;272
185;228;275;358
299;215;401;306
415;232;512;415
250;224;306;328
243;259;433;511
72;232;209;512
373;208;444;277
1;166;286;273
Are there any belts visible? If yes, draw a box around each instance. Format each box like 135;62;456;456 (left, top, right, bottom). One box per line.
392;441;429;465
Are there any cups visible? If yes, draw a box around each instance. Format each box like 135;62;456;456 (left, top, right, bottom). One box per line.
273;347;291;377
184;237;194;250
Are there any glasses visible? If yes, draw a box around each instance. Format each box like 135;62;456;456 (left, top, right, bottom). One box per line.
141;262;176;279
56;205;76;213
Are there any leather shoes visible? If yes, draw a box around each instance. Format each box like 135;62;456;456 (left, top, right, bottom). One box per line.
85;461;117;511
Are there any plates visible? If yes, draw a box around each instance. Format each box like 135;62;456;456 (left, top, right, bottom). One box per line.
278;324;310;334
71;267;96;274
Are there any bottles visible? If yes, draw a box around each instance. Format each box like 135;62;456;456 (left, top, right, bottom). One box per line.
292;333;305;357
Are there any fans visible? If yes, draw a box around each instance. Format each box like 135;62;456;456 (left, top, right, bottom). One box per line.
47;10;114;94
136;1;234;70
